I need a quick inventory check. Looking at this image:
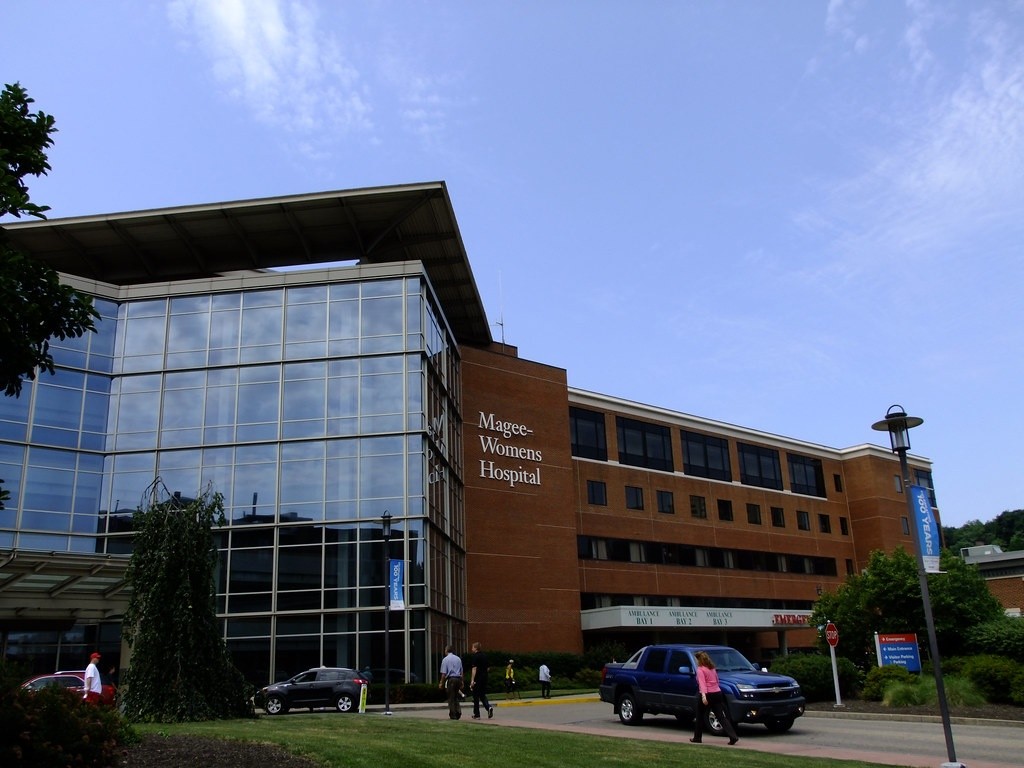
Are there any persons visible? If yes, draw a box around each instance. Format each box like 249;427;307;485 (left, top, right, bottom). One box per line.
539;662;551;698
363;666;372;680
690;652;739;745
83;653;102;704
105;667;117;690
506;660;514;697
470;642;493;719
438;645;464;719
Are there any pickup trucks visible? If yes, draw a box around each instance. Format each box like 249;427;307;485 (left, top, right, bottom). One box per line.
597;643;805;735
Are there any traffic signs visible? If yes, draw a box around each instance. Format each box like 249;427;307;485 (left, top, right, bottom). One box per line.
874;632;922;673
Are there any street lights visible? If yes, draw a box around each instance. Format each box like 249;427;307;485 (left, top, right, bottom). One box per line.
371;510;405;715
872;405;966;768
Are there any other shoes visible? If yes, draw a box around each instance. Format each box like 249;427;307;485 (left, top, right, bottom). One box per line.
450;712;461;720
488;707;494;718
472;715;480;720
728;738;738;745
690;738;702;743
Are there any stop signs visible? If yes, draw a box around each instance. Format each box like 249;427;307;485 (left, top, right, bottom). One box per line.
825;623;839;646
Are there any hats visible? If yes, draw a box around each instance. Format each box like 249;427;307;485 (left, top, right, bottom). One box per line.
91;653;100;658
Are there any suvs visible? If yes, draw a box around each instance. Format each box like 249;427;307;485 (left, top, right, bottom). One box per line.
252;666;371;715
15;670;117;706
360;667;420;685
